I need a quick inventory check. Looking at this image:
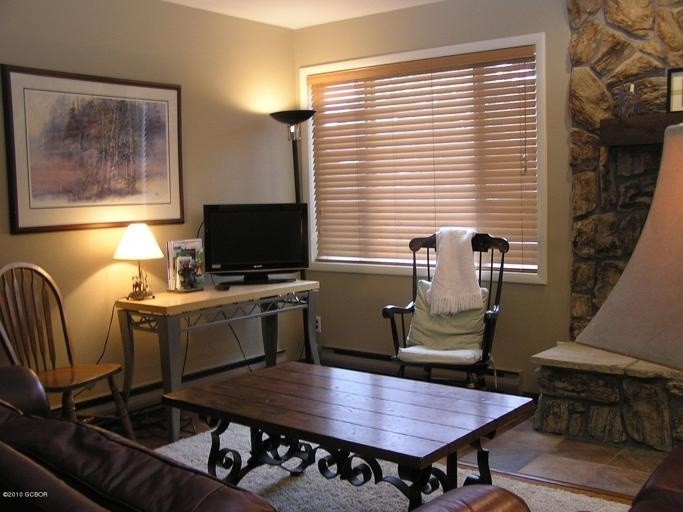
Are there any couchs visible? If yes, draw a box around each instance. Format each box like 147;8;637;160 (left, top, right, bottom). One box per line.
0;366;532;511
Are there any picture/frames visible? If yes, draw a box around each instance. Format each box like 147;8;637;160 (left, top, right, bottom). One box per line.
0;63;185;235
666;67;683;112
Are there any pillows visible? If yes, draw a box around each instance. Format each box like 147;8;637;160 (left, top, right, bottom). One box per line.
406;279;490;350
0;394;23;428
0;412;277;511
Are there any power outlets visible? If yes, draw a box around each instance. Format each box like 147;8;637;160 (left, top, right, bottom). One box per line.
314;315;321;334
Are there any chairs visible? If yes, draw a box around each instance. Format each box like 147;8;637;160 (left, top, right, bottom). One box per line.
0;261;136;440
382;231;510;388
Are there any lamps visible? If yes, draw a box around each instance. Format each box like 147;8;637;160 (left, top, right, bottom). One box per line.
270;109;317;363
113;222;165;296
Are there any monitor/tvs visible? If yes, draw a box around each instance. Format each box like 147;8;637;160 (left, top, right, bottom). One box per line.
203;203;310;284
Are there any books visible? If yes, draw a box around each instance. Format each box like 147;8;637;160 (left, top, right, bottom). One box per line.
165;238;205;292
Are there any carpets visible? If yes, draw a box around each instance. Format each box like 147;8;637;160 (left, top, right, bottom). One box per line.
152;420;638;512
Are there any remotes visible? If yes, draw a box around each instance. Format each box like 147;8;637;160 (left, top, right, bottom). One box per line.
215;285;230;290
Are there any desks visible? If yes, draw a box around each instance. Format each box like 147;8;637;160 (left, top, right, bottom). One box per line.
115;277;321;442
